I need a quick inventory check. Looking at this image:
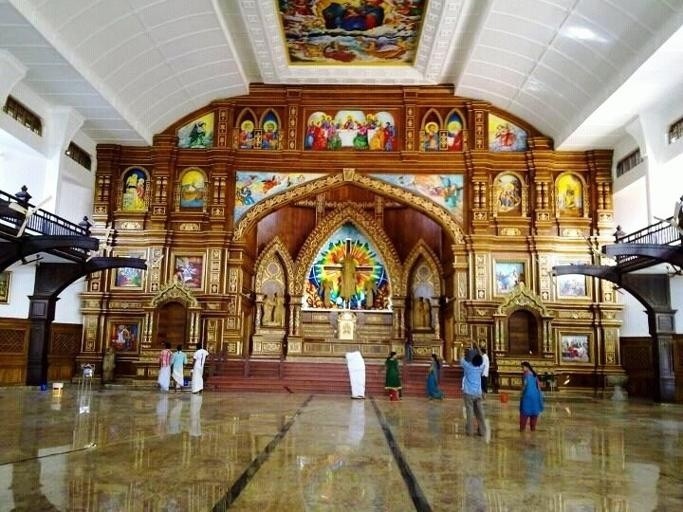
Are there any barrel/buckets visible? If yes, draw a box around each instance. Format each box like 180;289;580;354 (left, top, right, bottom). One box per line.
50;397;63;410
500;392;508;403
389;389;400;401
53;383;64;396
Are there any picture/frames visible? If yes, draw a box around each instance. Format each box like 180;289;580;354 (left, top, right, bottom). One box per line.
0;269;12;305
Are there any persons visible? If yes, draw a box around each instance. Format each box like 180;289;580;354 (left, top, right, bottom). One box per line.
414;298;430;328
385;352;402;400
158;395;203;441
261;292;285;323
481;349;490;399
190;343;210;393
157;343;173;392
282;1;422;62
366;280;373;307
426;354;445;400
324;279;331;307
171;344;188;393
457;340;486;436
520;362;544;432
